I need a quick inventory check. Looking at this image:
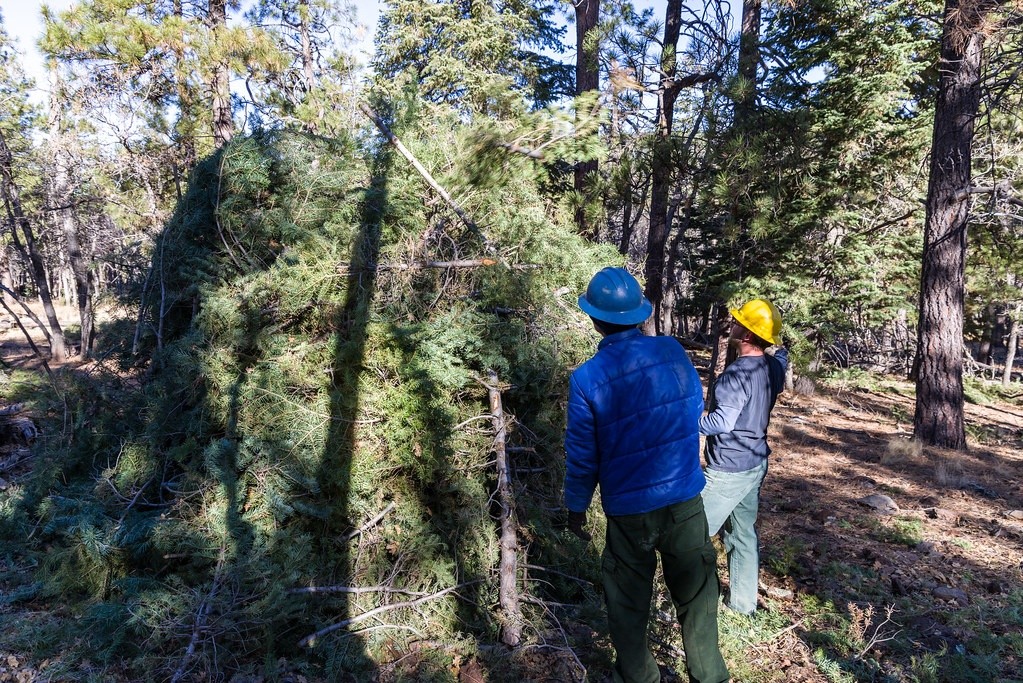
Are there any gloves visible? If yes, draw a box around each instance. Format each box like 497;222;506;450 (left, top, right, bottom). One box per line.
566;508;593;540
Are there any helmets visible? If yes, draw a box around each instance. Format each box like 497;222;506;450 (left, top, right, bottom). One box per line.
577;268;653;327
729;297;782;346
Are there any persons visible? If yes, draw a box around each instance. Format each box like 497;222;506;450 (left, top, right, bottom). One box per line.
700;295;791;612
564;267;730;683
